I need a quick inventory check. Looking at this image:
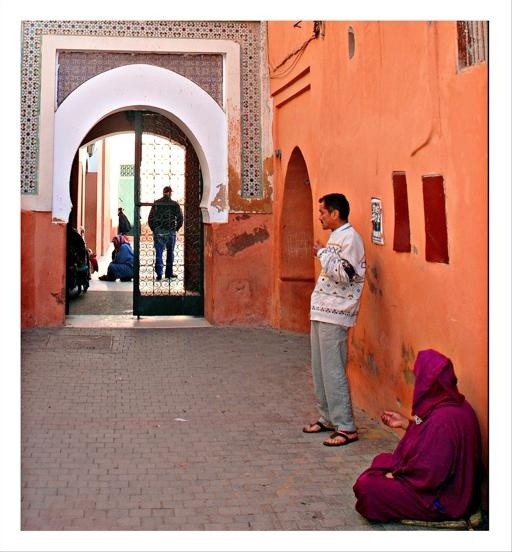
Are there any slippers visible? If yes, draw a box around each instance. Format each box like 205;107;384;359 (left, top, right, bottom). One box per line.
324;431;358;446
303;421;334;433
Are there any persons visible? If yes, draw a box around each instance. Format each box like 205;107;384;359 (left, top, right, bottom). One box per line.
352;350;482;524
302;193;366;447
148;184;184;280
66;222;99;294
117;208;131;235
98;235;134;283
